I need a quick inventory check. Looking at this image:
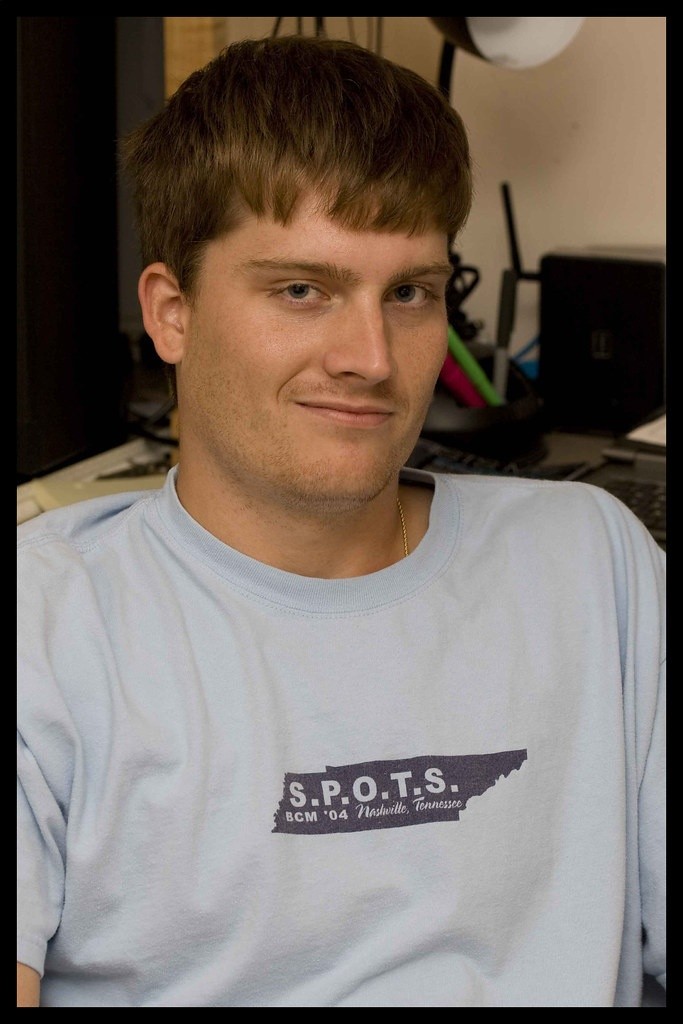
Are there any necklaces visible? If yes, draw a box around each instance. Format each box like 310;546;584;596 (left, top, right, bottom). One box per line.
396;496;408;558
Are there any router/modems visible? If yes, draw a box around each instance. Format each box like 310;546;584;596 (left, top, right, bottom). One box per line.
501;183;665;441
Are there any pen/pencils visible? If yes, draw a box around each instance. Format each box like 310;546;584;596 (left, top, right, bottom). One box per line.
442;267;520;410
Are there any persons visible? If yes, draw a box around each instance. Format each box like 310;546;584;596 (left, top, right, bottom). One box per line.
17;34;667;1006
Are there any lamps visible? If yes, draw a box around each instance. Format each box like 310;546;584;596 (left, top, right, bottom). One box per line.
417;17;584;461
